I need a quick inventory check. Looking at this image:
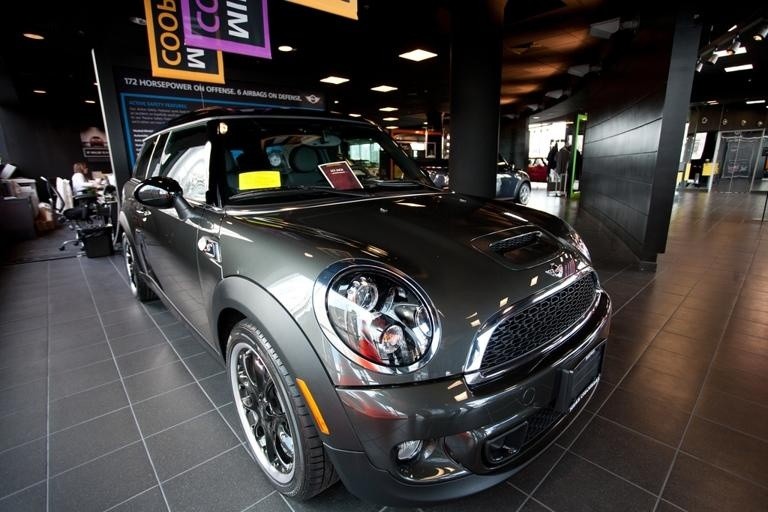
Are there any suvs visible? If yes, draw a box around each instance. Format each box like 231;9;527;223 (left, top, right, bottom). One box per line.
117;104;613;508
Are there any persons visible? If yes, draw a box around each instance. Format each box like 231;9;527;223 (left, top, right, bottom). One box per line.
71;160;105;195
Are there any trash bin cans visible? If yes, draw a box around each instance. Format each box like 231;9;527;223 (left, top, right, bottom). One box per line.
78;224;114;257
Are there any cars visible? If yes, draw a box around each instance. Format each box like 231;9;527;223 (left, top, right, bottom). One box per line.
351;159;380;177
416;154;533;206
527;157;553;183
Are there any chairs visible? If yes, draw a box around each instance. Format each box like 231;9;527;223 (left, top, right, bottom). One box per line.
227;140;325;189
59;184;117;256
